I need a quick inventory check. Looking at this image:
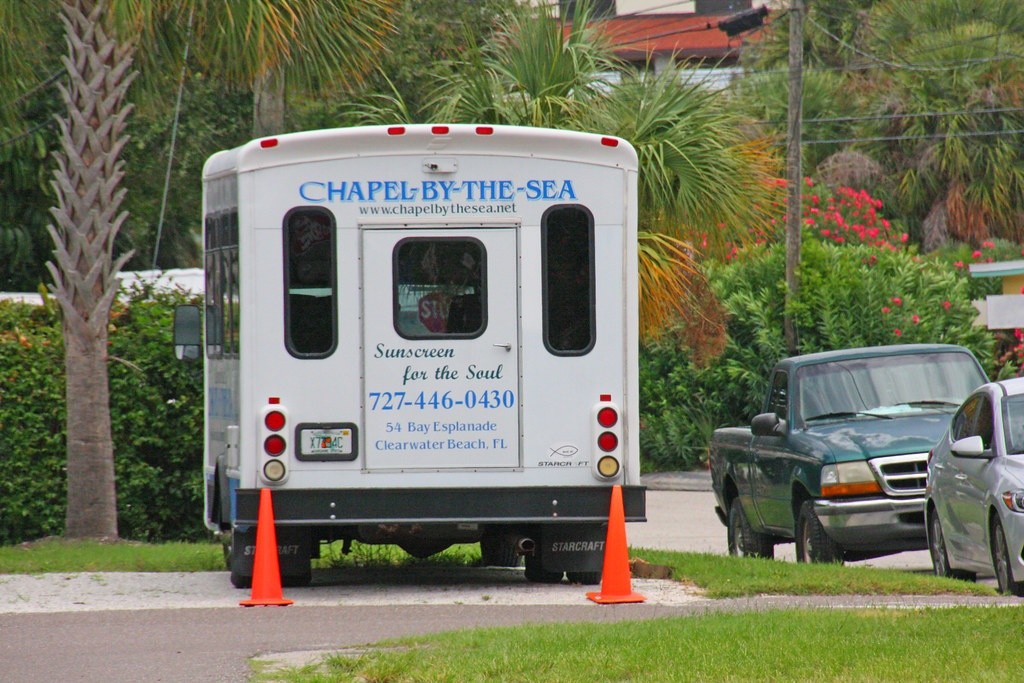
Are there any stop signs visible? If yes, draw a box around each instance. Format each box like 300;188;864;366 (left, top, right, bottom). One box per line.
419;292;453;333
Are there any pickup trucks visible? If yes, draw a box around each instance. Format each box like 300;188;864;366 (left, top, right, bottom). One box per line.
709;343;992;565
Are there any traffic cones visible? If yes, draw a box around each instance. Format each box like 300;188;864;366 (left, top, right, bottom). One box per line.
239;487;294;606
585;484;649;604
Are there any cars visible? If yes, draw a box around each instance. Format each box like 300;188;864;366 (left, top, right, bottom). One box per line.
924;376;1024;596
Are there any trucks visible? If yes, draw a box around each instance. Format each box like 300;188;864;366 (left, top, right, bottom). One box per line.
172;123;647;590
